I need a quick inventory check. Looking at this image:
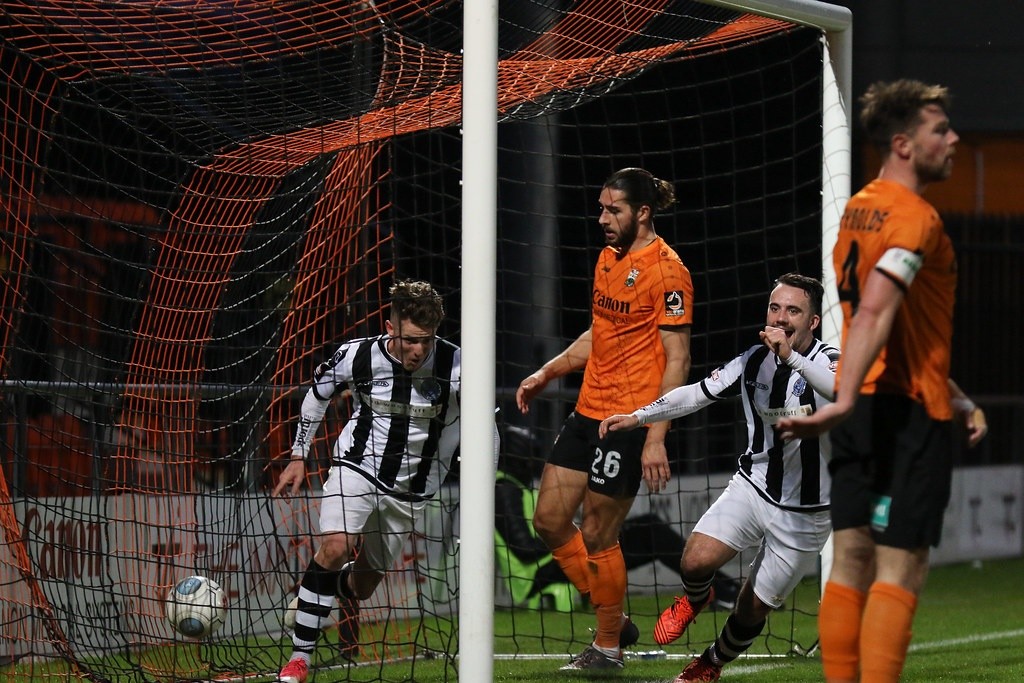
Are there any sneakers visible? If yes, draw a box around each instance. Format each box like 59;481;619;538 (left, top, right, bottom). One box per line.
652;584;715;647
557;644;625;671
276;657;307;683
672;657;721;683
568;619;640;664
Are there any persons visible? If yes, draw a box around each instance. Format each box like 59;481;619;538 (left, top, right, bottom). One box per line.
515;168;693;669
495;426;785;612
599;275;840;683
778;80;987;683
273;282;461;683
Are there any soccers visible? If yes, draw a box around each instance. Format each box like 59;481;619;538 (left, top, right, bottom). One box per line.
165;574;229;640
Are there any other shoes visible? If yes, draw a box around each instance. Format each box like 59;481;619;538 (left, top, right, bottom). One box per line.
284;596;298;627
